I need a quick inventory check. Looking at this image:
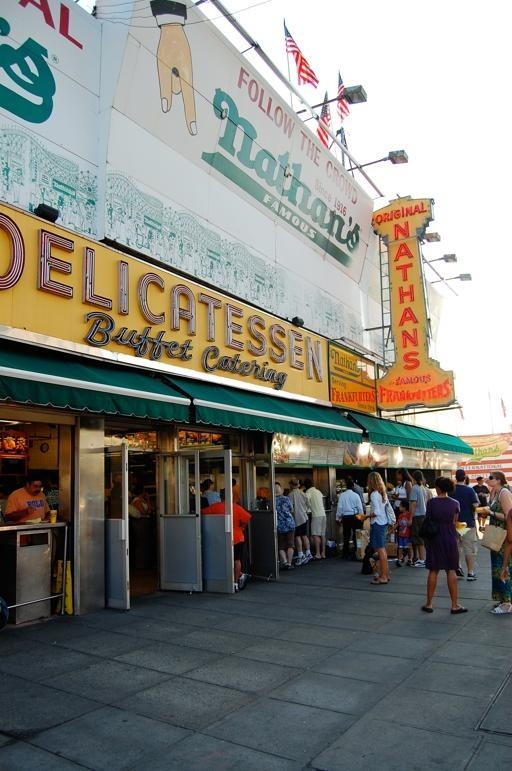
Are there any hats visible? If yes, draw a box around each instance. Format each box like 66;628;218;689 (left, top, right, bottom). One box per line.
275;484;285;496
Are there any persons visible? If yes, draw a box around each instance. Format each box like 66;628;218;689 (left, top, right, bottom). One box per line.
109;477;327;593
2;474;51;546
150;0;197;136
335;467;512;614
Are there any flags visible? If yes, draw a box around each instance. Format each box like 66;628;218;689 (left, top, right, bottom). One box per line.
285;24;319;89
317;91;331;148
336;70;350;124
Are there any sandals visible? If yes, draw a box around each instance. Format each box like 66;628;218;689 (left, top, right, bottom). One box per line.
370;575;391;585
489;601;512;615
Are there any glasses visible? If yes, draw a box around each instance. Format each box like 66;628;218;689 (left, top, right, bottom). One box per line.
489;476;498;480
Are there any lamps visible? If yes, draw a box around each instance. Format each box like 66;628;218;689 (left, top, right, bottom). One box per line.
422;253;459;266
348;146;408;180
424;231;440;243
429;271;472;284
295;84;366;118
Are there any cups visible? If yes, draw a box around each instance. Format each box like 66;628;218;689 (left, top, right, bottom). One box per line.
477;513;488;532
50;510;57;524
45;513;50;523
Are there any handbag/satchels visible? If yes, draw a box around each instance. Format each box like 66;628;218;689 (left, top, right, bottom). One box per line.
418;498;441;545
385;500;397;526
480;511;508;553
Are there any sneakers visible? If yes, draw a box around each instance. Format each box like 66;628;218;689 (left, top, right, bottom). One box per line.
456;565;480;582
421;605;434;614
395;557;426;568
234;572;252;594
281;552;326;570
450;603;468;615
